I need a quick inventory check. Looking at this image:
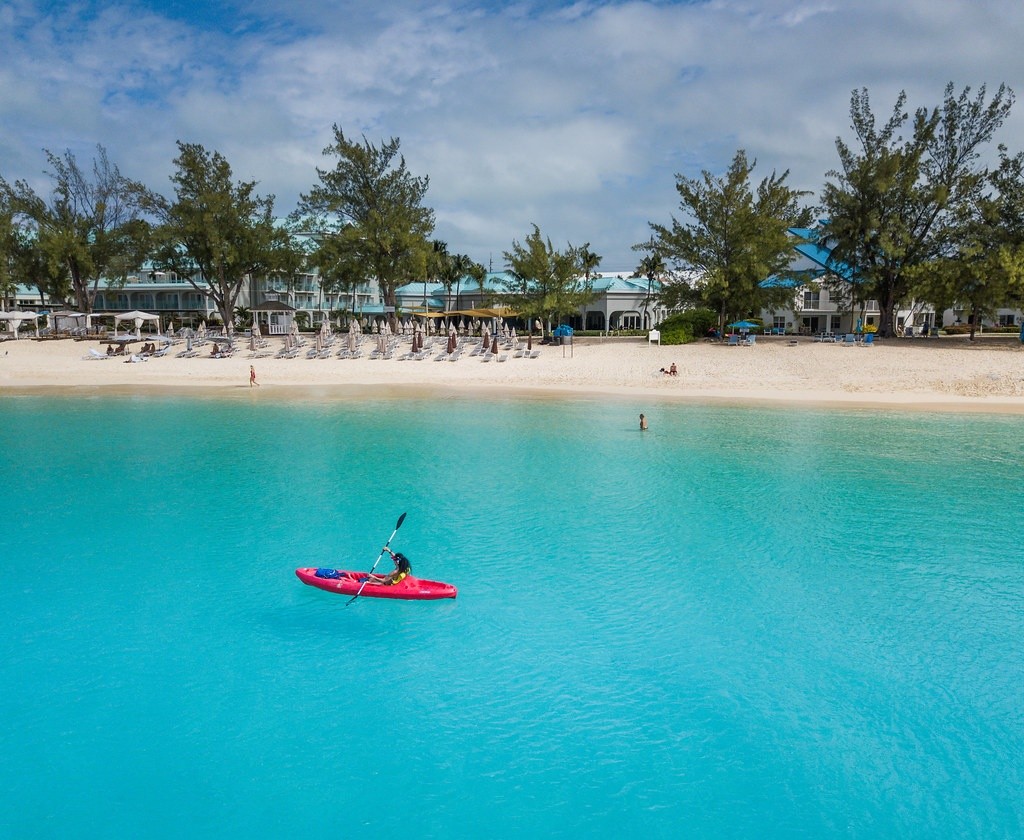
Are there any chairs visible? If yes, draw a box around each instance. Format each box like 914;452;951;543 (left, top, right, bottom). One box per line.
863;334;874;347
778;328;786;336
743;335;756;346
530;351;543;358
929;327;940;339
22;325;107;336
905;327;915;338
834;335;844;343
790;334;799;347
844;334;855;347
764;329;771;335
728;335;738;346
108;333;514;362
515;343;525;350
814;333;822;342
514;350;524;358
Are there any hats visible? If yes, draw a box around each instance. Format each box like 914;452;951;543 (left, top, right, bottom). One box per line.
391;553;403;559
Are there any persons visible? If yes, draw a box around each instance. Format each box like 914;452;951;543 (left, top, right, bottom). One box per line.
249;365;261;388
368;546;413;586
920;320;929;337
107;341;157;355
658;363;678;377
639;413;648;430
210;343;234;359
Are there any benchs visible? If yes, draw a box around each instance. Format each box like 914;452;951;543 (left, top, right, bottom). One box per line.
861;335;881;342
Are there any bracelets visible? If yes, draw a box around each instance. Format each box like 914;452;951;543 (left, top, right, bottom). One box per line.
389;550;392;554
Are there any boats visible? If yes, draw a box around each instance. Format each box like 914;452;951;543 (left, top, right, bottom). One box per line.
295;567;457;600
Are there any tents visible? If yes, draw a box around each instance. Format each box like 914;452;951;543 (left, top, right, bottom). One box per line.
0;311;162;343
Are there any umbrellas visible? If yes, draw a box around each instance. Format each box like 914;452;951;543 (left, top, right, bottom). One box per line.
165;304;546;359
855;318;863;343
145;334;168;351
115;334;139;353
728;321;759;340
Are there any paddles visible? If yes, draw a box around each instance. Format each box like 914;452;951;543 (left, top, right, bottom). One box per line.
344;511;408;608
